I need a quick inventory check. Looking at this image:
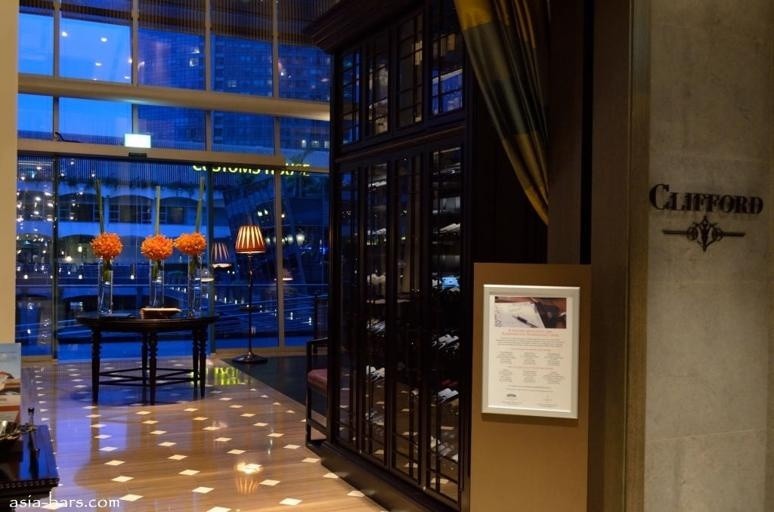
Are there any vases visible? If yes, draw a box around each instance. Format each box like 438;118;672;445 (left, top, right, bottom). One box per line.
148;256;203;309
96;259;112;314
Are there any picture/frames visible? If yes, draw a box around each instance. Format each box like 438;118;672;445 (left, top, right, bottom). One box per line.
482;283;579;420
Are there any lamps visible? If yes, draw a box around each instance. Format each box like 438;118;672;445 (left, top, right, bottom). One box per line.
234;223;266;365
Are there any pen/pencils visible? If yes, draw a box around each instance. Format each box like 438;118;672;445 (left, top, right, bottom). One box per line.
513;315;538;328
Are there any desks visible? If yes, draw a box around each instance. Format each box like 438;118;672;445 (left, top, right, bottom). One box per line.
76;309;219;404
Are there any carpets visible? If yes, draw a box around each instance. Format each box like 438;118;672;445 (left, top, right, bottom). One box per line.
221;354;328;418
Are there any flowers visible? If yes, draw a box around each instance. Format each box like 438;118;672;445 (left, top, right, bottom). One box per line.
90;232;122;286
140;233;206;281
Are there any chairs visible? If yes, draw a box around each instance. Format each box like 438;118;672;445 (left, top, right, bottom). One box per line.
305;343;349;449
